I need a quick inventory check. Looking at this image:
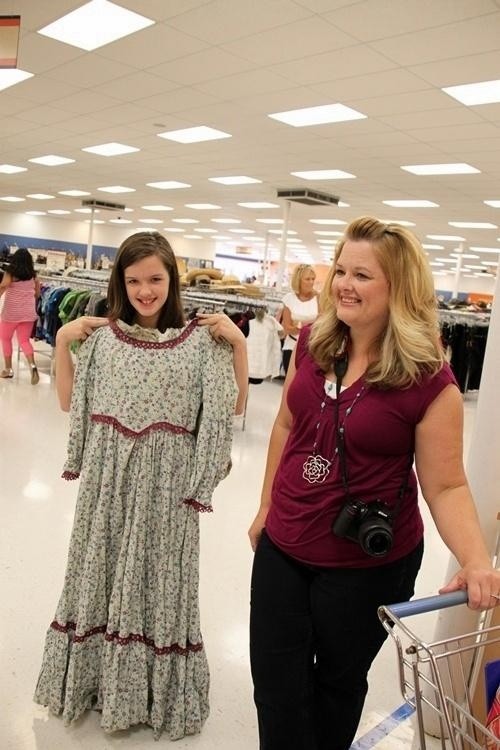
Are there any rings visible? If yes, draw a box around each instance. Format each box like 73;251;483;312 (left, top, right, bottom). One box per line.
490;593;500;600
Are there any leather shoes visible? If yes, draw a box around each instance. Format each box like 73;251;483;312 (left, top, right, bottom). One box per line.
0;368;13;378
31;364;39;385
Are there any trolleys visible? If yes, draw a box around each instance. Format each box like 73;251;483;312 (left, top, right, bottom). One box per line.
377;568;500;750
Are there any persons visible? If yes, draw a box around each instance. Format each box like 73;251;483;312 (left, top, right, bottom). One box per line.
0;247;41;384
281;261;324;376
53;231;250;741
245;218;500;750
